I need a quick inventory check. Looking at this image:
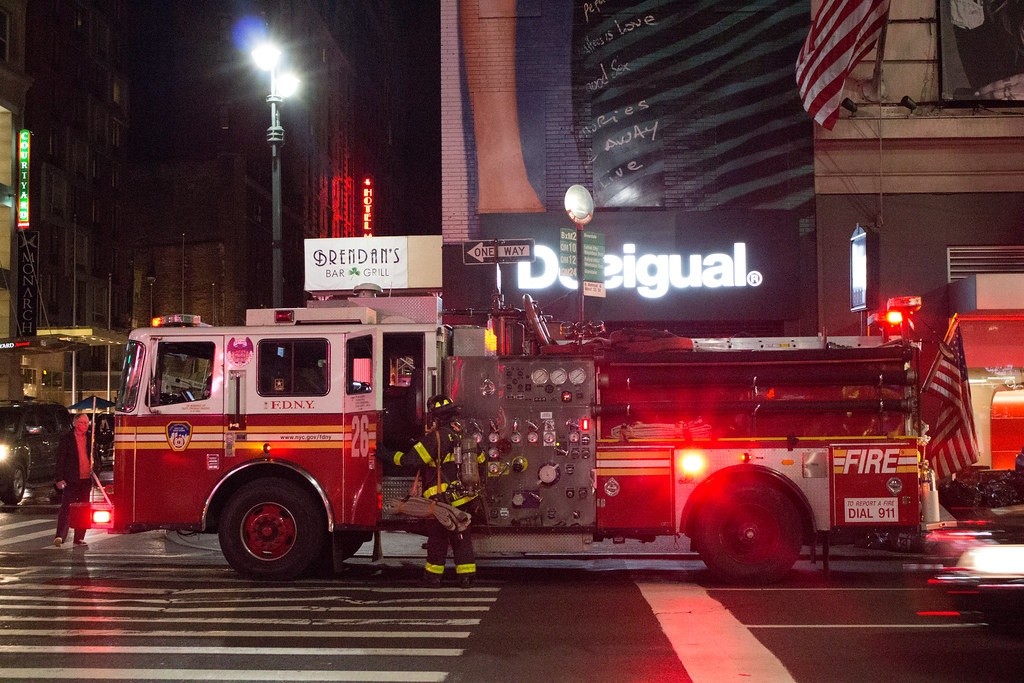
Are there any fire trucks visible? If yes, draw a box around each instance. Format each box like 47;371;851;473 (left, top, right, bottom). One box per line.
76;284;956;585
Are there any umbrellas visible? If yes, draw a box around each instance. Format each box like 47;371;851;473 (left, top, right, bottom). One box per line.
66;393;116;411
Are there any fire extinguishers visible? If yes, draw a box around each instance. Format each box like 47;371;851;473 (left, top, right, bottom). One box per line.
918;459;940;523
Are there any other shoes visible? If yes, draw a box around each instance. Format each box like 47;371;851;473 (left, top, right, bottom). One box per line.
461;573;476;587
422;569;442;587
53;537;63;547
72;539;88;546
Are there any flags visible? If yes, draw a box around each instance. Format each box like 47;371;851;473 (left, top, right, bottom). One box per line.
794;0;890;132
921;322;982;486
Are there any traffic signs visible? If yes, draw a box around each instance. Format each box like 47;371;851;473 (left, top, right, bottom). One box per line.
461;238;536;266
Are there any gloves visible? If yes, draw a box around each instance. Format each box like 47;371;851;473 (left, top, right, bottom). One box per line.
376;443;397;464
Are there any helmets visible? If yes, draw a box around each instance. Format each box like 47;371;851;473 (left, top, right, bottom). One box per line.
428;394;459;415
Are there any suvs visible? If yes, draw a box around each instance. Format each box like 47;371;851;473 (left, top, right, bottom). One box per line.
0;399;74;506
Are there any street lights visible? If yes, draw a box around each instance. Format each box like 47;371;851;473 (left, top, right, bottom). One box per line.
252;41;300;308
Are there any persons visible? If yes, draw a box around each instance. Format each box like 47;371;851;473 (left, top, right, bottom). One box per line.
52;413;96;547
372;394;485;590
